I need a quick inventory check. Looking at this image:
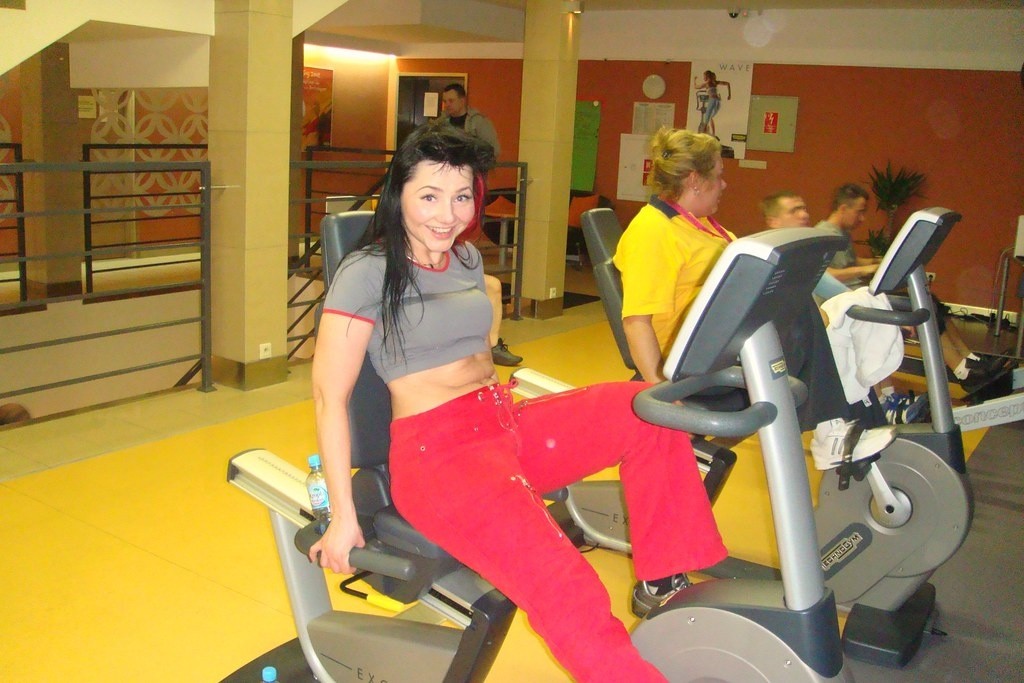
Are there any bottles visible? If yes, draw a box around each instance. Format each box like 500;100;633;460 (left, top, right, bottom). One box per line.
306;453;331;535
262;666;279;683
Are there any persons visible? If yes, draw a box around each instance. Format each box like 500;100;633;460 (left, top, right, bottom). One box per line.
432;84;500;159
813;184;1021;395
308;113;730;683
483;274;524;367
763;190;931;425
610;124;902;469
693;70;731;137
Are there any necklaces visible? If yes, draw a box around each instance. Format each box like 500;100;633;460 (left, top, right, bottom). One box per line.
421;253;444;269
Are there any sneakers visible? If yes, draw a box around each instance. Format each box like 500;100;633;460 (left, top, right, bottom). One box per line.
958;355;1008;393
810;423;897;471
891;391;929;406
880;396;923;425
491;338;523;366
632;572;693;620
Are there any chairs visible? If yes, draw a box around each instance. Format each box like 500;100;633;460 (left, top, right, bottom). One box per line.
476;187;519;248
567;189;614;266
